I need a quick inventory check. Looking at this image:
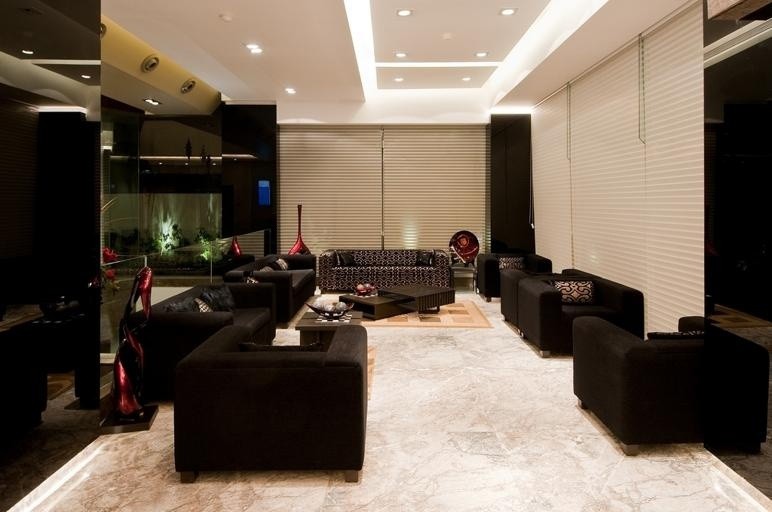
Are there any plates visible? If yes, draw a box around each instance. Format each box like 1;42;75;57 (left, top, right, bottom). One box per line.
449;230;479;264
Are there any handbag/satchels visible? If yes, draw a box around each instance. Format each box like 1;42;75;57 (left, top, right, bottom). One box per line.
202;286;236;311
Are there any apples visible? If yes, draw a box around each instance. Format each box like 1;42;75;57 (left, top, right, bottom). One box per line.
356;283;375;290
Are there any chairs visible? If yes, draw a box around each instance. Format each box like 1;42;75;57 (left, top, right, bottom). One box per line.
174;322;367;483
